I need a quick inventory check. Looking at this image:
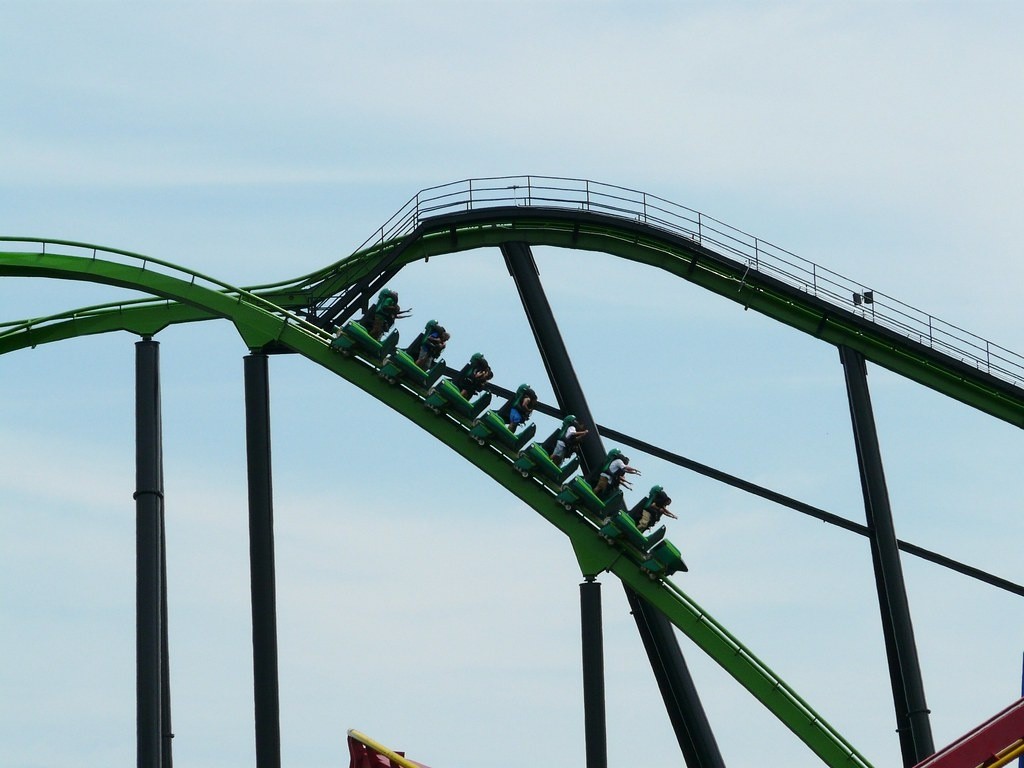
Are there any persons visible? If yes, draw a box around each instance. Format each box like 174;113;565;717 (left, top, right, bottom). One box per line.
371;288;679;531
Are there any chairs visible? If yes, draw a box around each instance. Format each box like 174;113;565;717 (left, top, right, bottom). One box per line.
342;289;669;550
